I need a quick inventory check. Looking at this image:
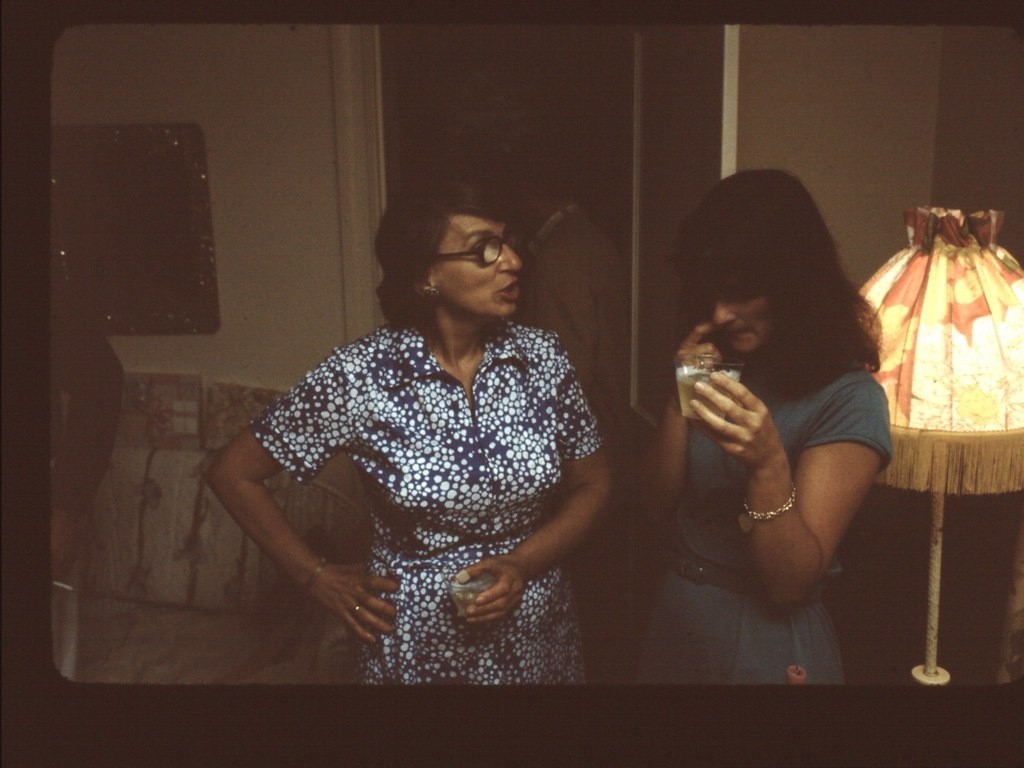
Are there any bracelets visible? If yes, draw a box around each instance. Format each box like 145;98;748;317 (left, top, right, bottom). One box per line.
744;477;799;524
305;560;326;591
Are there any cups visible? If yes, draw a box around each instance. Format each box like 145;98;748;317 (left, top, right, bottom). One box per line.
667;354;745;420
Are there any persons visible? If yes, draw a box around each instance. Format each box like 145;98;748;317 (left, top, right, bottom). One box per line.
202;178;616;685
629;167;896;685
47;254;126;555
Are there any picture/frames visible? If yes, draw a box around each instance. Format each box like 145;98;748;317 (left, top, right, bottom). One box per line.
48;119;226;335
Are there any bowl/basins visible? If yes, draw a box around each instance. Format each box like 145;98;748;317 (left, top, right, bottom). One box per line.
449;572;498;621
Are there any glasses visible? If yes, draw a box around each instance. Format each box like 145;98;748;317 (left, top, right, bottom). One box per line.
427;217;522;267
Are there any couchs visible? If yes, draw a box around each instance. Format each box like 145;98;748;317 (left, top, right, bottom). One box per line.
50;372;396;688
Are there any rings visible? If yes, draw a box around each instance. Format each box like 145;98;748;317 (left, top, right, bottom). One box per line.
352;604;359;616
696;353;705;367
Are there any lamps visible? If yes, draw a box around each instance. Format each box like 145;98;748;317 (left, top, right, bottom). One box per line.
855;203;1024;687
